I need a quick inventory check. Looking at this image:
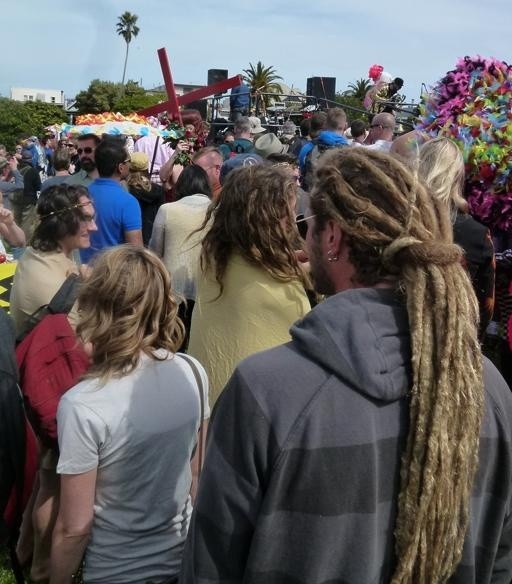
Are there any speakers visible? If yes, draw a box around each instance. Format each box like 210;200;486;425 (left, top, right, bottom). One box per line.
307;76;335;109
185;100;207;121
208;69;228;93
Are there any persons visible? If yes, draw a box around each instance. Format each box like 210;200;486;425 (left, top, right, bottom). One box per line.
48;244;211;584
0;107;497;416
178;147;512;584
9;183;98;583
371;78;406;123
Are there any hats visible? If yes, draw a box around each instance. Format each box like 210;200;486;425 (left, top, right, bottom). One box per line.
0;157;9;168
248;133;289;160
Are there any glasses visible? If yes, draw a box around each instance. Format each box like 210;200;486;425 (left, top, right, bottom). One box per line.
77;148;95;155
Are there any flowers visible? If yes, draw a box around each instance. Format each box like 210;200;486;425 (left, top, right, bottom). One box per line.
369;63;384;83
162;116;211;166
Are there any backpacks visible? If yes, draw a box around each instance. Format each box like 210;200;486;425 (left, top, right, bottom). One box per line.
15;273;95;452
300;139;335;192
